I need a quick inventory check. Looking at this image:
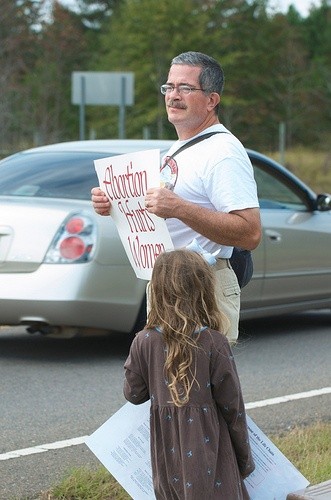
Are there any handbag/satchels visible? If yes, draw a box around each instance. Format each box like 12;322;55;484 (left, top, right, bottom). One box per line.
229;250;253;288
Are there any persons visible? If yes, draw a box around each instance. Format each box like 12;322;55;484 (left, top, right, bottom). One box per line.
123;248;256;500
91;51;262;349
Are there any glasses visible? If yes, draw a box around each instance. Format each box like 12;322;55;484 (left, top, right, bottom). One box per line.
161;85;204;96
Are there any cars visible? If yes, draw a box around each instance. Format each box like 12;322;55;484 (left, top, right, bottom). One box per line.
0;137;331;342
